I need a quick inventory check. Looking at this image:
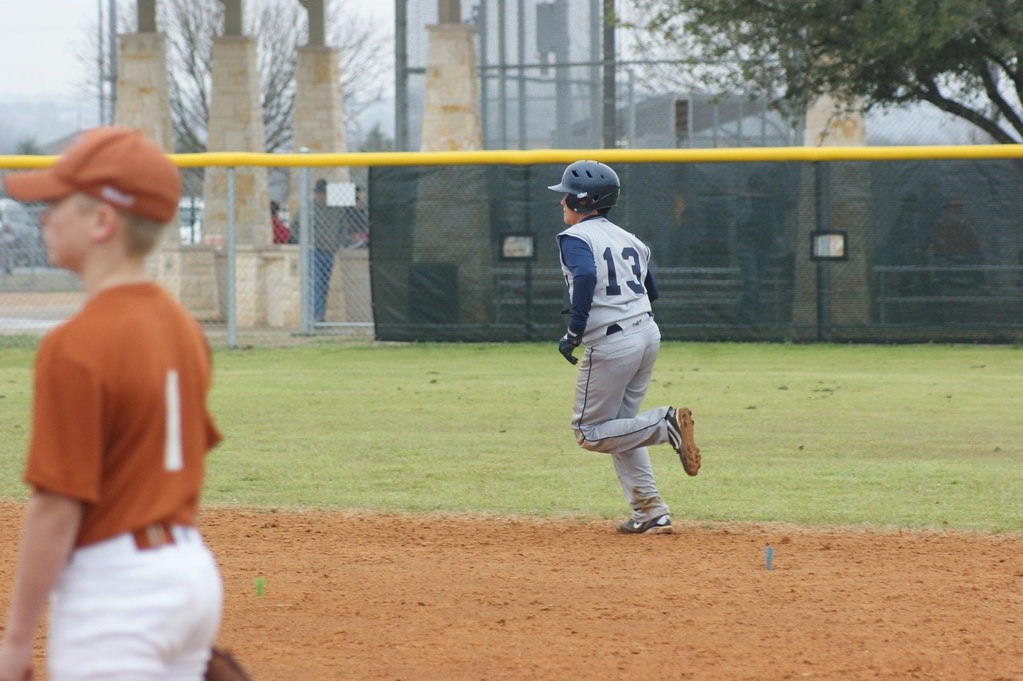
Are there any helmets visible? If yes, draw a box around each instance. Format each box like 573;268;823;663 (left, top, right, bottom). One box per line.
547;160;621;214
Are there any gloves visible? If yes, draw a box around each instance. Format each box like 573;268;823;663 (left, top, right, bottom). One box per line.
559;326;582;365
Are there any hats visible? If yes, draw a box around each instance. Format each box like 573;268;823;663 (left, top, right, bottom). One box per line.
3;125;182;223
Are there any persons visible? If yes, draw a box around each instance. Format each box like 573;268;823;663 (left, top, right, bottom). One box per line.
547;160;702;534
0;126;224;681
291;179;346;330
344;185;369;250
270;201;291;244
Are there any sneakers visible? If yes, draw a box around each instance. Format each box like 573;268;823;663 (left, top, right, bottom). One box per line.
664;406;702;476
616;513;673;535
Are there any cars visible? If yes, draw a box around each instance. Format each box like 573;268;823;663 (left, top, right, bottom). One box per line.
0;198;56;277
177;196;205;245
